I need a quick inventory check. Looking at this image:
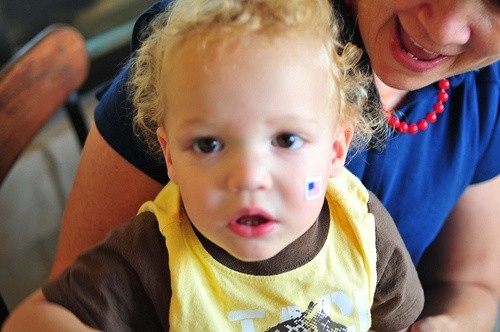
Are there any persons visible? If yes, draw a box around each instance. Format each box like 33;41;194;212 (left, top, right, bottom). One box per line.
47;0;500;332
0;0;425;332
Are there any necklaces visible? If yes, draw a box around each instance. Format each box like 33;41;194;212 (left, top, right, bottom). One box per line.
380;77;449;135
